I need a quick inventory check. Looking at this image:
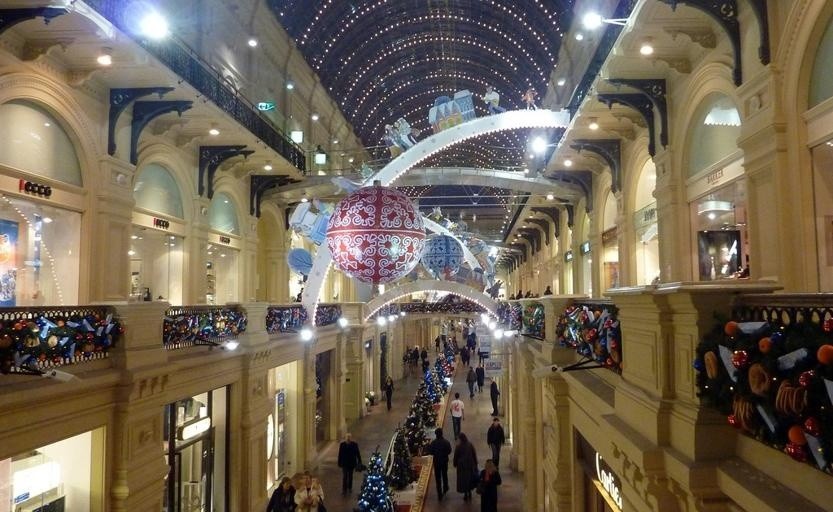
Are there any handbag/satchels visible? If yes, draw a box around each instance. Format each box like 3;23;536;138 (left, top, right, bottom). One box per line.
318;503;326;512
476;479;483;494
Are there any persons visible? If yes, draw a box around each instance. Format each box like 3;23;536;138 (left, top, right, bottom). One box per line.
452;430;477;500
265;475;297;512
382;375;394;411
489;378;499;416
485;417;504;470
293;473;324;512
401;332;486;399
474;458;501;512
449;391;464;441
336;433;361;496
429;427;451;502
509;285;552;301
